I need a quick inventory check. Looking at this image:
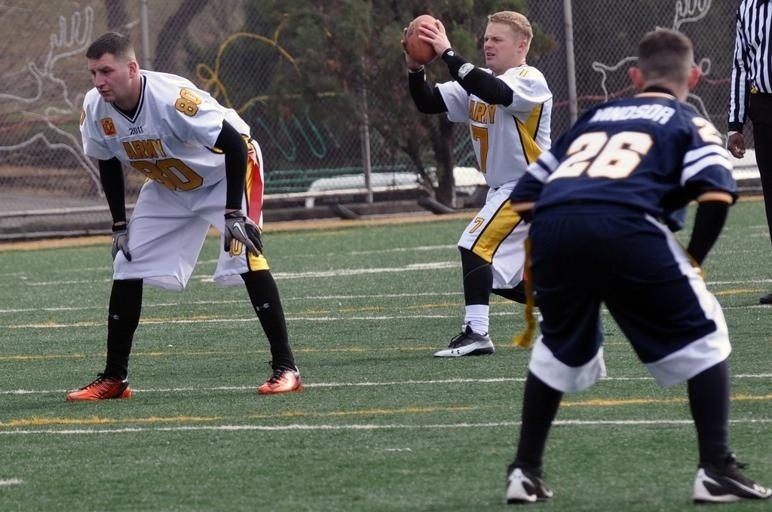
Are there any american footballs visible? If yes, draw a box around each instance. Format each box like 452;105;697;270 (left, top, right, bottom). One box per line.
406;15;438;65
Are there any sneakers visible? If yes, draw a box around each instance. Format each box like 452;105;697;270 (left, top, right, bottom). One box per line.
66;373;131;401
258;365;301;394
506;468;552;504
760;294;772;305
434;325;491;356
693;453;772;503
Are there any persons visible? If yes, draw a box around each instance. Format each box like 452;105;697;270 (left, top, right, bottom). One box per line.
398;7;561;360
504;19;772;508
63;28;305;403
725;1;772;306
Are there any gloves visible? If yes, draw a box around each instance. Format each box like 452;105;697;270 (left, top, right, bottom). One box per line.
224;210;264;257
111;224;132;262
